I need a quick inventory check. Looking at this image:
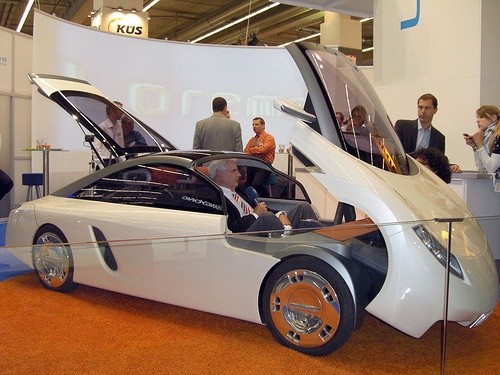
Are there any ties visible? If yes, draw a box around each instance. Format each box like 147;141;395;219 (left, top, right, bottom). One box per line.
232;193;251;215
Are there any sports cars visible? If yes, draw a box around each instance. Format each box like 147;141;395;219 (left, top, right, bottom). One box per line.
4;40;500;357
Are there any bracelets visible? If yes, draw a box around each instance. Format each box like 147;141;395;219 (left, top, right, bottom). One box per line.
476;144;483;149
281;212;285;215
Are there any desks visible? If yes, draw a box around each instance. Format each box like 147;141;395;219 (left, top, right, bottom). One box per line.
275;152;293;199
22;149;71;198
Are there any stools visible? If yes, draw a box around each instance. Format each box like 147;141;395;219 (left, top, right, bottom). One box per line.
21;173;43;202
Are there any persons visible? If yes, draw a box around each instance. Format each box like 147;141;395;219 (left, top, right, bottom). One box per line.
395;94;464;173
336;104;378;136
225;111;230;119
208;158;320;237
411;147;451;185
94;99;146;172
464;105;500;194
242;117;276;166
193;97;243;152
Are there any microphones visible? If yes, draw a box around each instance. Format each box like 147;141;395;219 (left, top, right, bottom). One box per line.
246;186;260;207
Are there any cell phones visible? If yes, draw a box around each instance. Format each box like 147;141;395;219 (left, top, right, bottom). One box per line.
464;133;472;140
